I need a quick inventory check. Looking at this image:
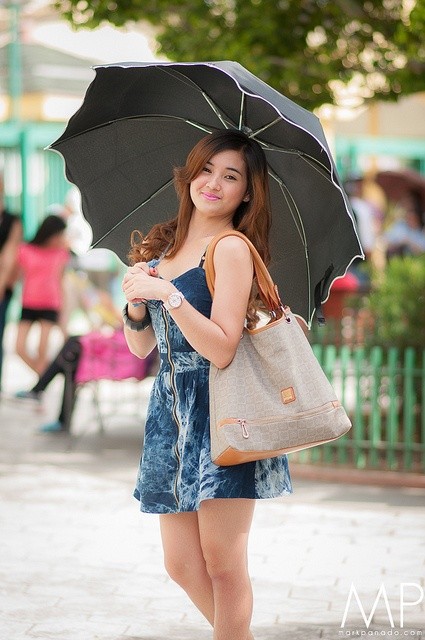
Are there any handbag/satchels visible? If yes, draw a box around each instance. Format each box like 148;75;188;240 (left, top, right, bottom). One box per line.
208;307;352;467
76;332;148;382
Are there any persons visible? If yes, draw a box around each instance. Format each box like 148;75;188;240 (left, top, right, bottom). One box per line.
385;183;425;258
0;174;22;394
16;303;159;433
122;128;294;639
16;214;72;380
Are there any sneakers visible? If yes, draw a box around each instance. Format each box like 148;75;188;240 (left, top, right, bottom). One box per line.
16;390;38;398
43;421;67;433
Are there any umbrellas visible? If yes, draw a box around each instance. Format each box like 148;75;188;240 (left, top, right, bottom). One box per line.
41;59;366;331
374;169;425;204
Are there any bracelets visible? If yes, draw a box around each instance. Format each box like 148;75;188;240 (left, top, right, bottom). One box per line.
121;304;151;332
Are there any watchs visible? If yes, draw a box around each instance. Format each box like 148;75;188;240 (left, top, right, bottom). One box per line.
162;291;184;310
